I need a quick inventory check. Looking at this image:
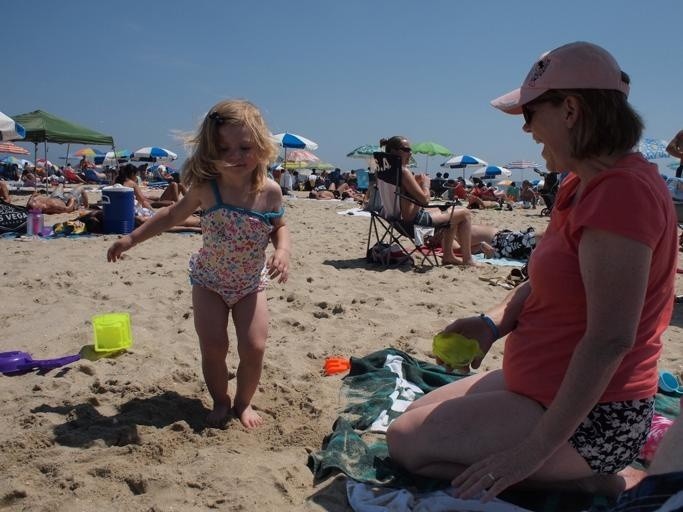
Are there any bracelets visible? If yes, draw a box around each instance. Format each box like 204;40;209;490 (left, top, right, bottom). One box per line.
480;314;500;340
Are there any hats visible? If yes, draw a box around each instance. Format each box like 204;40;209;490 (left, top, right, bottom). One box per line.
490;41;629;114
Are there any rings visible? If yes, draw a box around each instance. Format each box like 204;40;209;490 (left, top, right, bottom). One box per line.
486;473;496;482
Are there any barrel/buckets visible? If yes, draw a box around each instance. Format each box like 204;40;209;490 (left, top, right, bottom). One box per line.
102;187;135;235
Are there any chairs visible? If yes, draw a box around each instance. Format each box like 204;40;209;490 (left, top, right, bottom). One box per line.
365;152;457;267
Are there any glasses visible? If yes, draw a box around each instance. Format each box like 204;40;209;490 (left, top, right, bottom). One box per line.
521;94;555;123
397;147;411;153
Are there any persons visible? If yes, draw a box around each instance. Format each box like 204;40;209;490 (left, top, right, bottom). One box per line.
0;155;201;226
429;170;560;212
106;97;291;431
665;128;683;177
288;168;356;200
384;39;679;503
378;134;483;266
423;225;544;258
